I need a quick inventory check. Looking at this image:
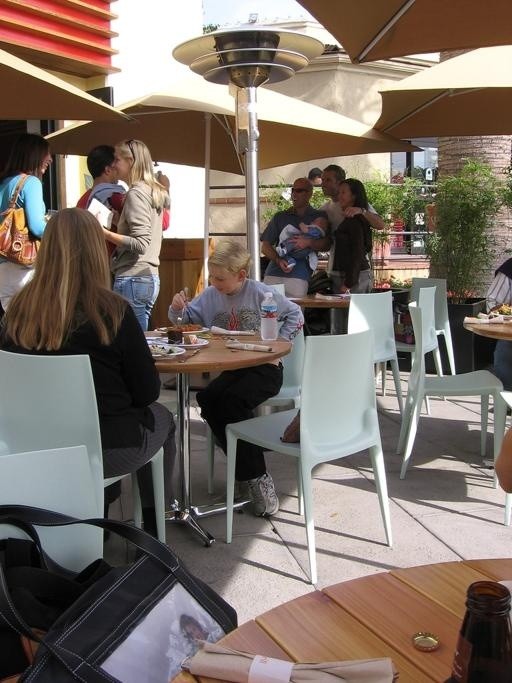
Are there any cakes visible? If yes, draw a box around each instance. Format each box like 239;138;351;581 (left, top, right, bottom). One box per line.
167;329;184;343
184;335;197;343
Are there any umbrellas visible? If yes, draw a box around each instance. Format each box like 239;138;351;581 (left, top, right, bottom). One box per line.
46;79;424;288
297;0;512;66
1;47;142;126
368;43;512;139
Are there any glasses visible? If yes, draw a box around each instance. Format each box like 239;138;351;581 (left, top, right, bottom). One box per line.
125;139;136;163
292;188;312;193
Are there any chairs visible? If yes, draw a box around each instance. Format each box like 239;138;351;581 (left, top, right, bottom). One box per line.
0;349;166;544
348;290;403;416
381;286;446;415
396;302;506;488
207;326;305;515
412;278;456;375
500;391;512;525
226;324;393;584
0;445;104;573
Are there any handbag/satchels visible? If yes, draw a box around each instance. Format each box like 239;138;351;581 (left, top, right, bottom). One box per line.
0;504;240;683
0;172;40;266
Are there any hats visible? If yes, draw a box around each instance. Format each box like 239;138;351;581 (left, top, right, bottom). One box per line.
308;168;322;177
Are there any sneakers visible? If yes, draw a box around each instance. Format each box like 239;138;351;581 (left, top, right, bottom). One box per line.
248;470;280;518
235;480;249;514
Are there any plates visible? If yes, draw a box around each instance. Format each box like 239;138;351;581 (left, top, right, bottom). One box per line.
494;314;512;320
153;336;209;350
148;345;186;361
154;326;210;335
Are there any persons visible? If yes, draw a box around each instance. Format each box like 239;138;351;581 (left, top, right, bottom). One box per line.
1;130;54;313
481;255;511;391
179;613;210;643
0;208;179;561
94;136;166;332
278;167;322;212
493;426;511;495
331;179;372;335
316;164;386;334
166;238;307;517
75;143;172;259
259;177;333;313
275;216;331;268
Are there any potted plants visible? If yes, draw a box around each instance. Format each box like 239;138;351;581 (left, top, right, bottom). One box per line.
363;169;423;304
424;158;507;376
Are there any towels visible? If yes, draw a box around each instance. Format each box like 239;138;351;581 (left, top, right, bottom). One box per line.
464;316;505;324
210;325;256;336
224;337;273;352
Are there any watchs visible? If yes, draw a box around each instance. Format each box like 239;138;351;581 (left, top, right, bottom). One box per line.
360;207;367;215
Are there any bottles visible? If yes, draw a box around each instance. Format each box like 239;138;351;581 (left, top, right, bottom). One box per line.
442;580;512;683
260;292;278;340
